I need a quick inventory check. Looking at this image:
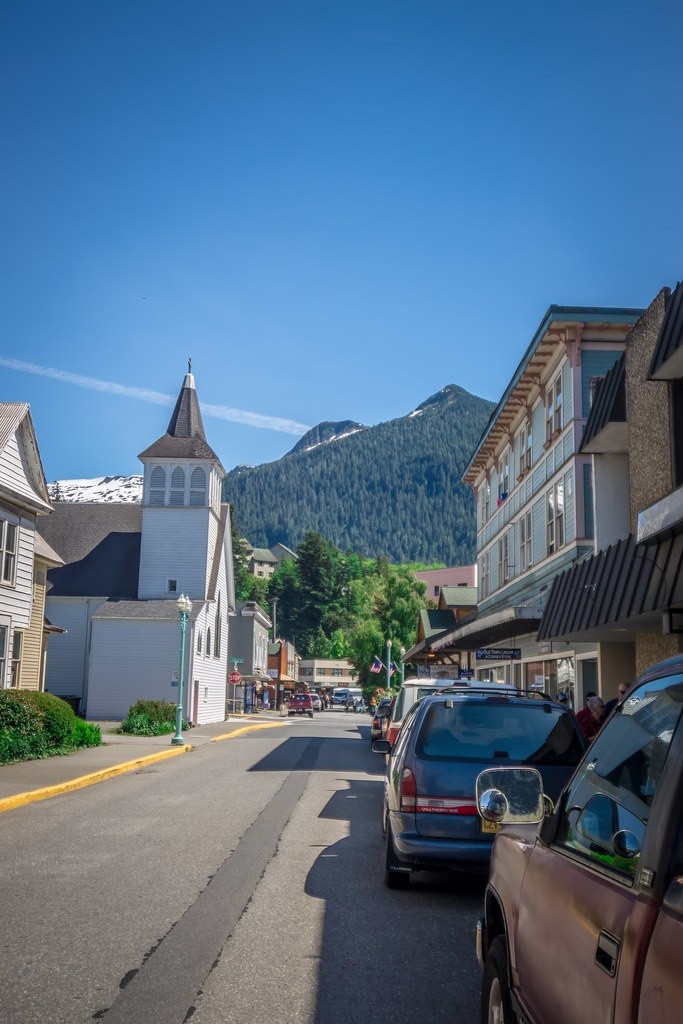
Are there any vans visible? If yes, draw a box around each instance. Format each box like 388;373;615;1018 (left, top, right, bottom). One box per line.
331;688;363;705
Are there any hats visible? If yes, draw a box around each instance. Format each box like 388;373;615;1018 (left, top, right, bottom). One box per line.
557;694;568;701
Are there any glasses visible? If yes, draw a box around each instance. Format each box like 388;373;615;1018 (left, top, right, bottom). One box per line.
598;705;604;709
621;690;626;694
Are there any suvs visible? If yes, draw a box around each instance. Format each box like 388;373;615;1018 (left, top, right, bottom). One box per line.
371;688;589;898
377;678;520;769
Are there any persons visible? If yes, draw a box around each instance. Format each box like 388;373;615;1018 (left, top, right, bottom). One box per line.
579;696;606;745
603;681;647;804
323;692;376;717
555;693;575;718
577;692;597;726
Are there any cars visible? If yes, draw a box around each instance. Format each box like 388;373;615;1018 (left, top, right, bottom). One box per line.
477;654;682;1024
370;699;395;748
287;694;314;718
307;694;322;713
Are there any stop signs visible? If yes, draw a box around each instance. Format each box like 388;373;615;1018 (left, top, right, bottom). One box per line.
228;671;242;685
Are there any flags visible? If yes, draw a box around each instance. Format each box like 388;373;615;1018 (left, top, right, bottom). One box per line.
370;659;382;674
388;663;396;676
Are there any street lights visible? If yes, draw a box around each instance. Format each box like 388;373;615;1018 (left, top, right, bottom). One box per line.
386;639;393;690
171;592;193;746
